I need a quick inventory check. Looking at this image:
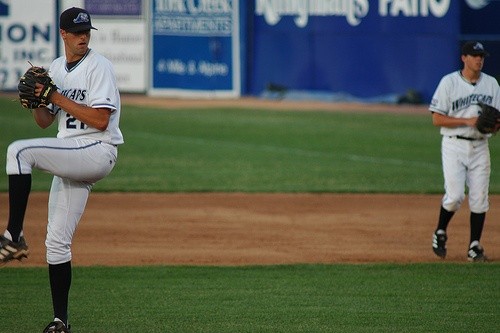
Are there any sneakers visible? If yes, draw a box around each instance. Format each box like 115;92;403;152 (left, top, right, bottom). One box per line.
433;228;447;257
0;235;30;267
43;321;71;333
467;246;487;262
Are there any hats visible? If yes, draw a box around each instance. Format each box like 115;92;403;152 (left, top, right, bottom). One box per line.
462;42;489;56
60;7;98;33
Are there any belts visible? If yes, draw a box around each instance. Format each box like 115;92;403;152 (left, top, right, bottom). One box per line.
457;136;483;140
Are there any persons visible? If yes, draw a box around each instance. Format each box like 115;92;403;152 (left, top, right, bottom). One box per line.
428;40;500;263
0;8;124;333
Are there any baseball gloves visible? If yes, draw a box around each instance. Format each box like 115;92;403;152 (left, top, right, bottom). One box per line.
18;64;56;111
476;100;499;133
